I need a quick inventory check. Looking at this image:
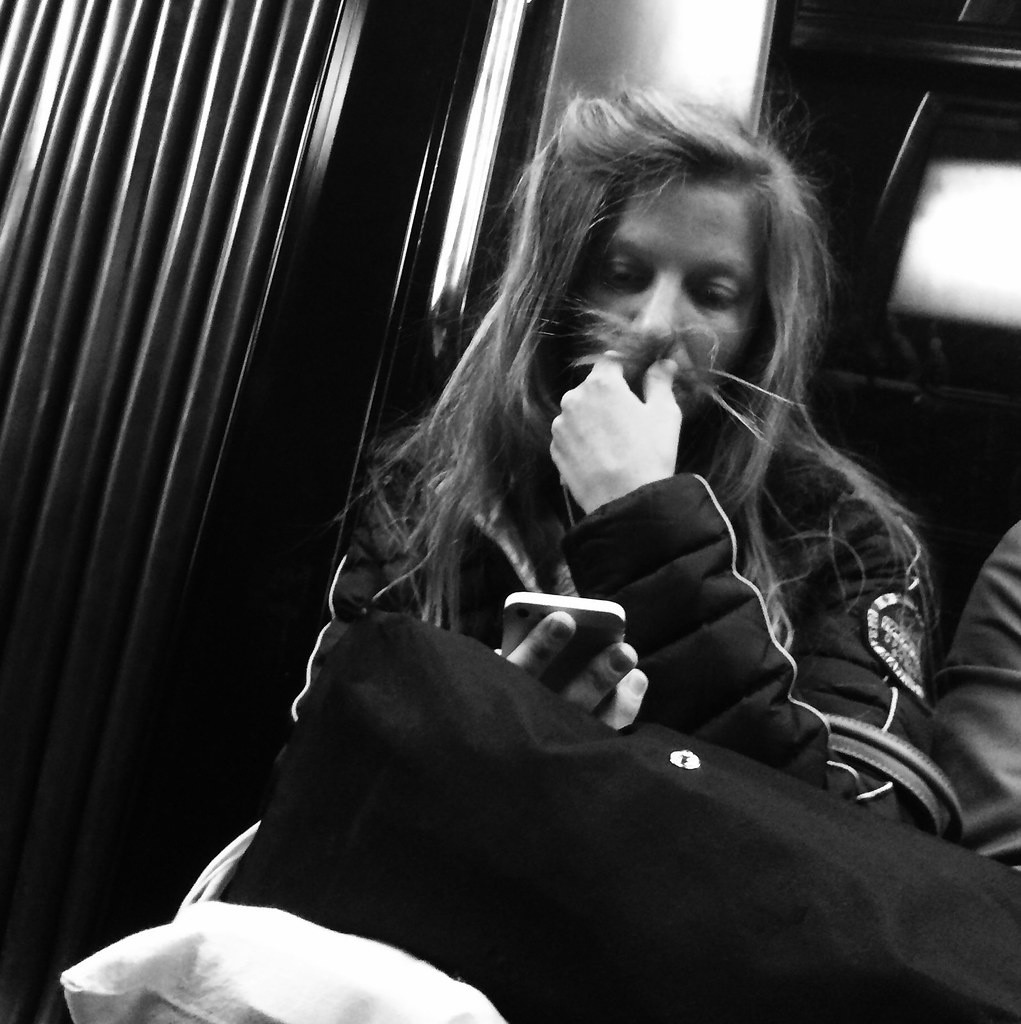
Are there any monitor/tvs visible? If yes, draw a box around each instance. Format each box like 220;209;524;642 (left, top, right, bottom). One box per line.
854;89;1021;409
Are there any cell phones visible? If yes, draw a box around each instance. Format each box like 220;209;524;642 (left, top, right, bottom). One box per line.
502;593;626;695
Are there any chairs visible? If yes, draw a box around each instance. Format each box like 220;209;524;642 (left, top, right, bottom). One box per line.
814;367;1021;636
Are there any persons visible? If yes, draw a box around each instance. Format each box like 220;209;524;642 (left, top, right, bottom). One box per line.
220;88;941;834
